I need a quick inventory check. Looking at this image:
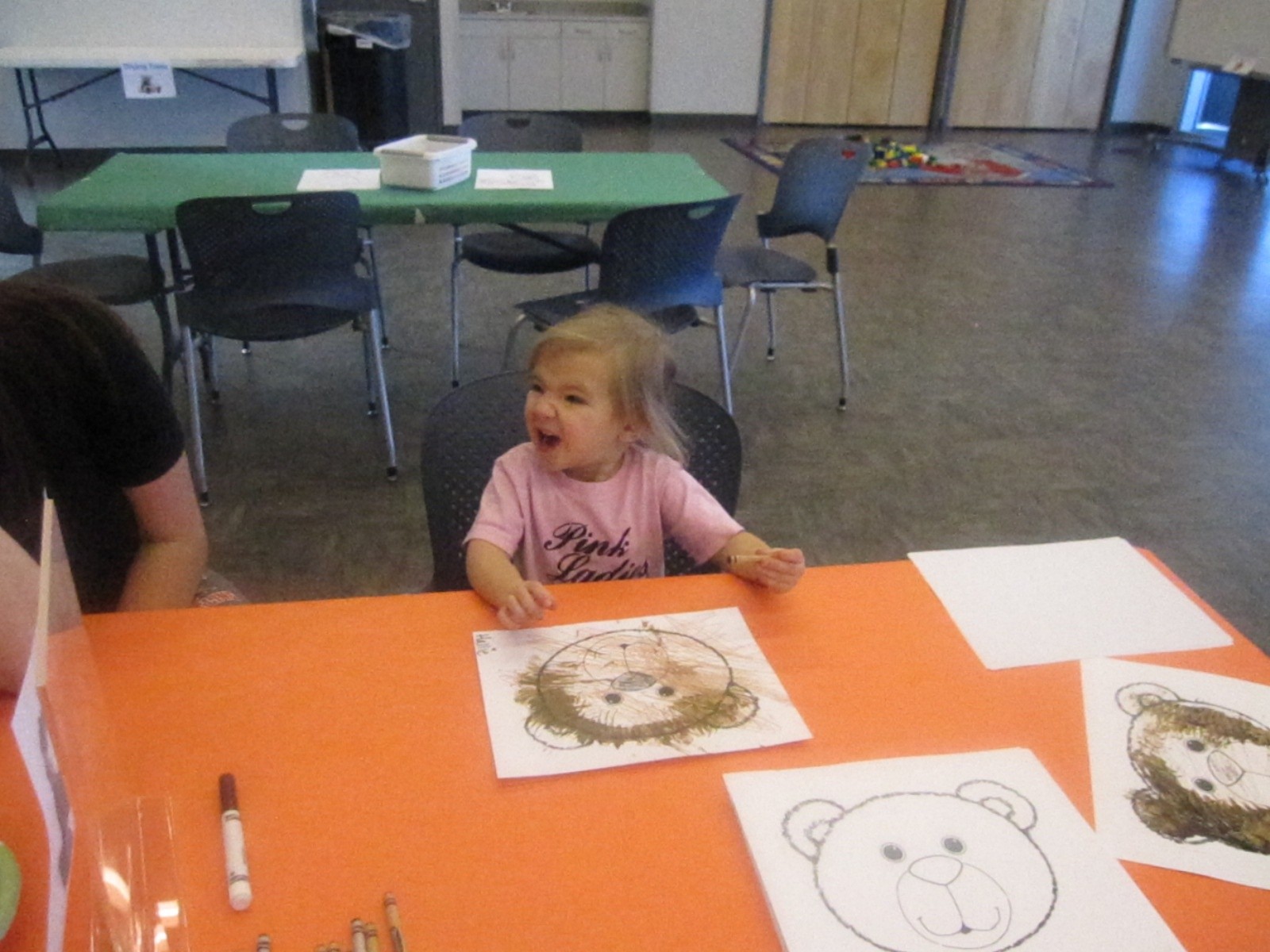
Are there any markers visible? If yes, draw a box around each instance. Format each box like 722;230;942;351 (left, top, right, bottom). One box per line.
220;773;252;911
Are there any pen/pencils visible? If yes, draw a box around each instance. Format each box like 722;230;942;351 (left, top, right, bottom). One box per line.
385;892;404;952
351;918;367;952
365;921;380;952
258;934;270;952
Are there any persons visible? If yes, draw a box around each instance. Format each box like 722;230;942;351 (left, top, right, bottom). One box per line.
0;278;249;699
462;303;805;630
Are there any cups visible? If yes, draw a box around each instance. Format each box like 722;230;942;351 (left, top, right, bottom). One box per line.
495;0;512;14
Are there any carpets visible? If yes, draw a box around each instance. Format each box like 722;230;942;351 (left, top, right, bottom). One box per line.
722;137;1113;188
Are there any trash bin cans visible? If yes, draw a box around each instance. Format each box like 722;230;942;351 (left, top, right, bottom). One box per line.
318;10;410;153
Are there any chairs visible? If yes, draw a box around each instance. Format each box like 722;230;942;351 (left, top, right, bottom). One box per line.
500;195;745;415
416;373;741;590
717;137;868;410
0;181;171;381
450;113;603;386
175;190;399;508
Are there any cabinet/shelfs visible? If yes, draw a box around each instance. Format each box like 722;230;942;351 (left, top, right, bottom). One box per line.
459;18;650;111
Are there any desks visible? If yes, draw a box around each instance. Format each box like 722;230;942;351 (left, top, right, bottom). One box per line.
0;47;300;188
36;151;730;385
0;537;1269;952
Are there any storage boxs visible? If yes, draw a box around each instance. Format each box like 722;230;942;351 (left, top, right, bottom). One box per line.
372;134;477;192
